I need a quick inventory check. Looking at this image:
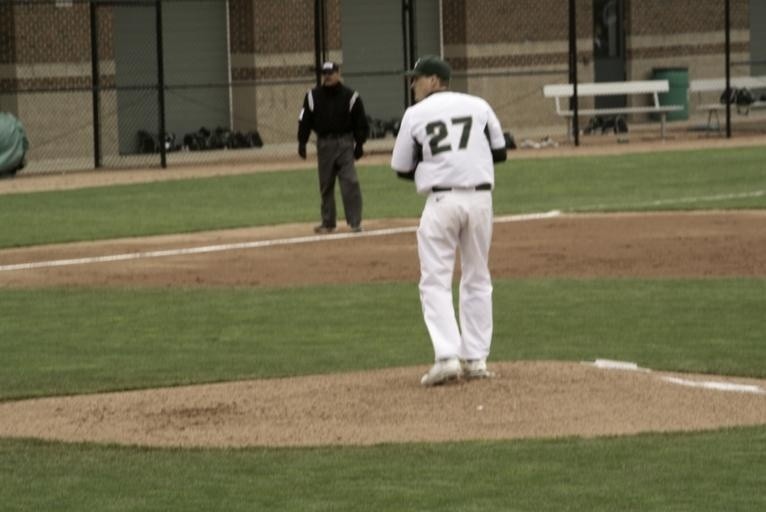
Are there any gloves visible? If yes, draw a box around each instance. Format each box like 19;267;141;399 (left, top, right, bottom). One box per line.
354;144;363;159
298;144;307;158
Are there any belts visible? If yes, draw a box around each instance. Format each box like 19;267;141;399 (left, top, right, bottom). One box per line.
432;184;491;190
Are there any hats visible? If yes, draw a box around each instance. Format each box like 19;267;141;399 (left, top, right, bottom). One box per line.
319;62;338;75
400;55;450;78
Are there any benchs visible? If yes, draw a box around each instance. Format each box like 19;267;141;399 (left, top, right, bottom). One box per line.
544;80;685;140
690;76;766;139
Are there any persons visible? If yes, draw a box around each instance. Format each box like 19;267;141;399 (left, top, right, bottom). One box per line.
390;55;507;386
297;61;368;235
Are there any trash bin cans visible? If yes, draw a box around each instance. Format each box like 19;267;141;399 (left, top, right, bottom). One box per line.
652;67;690;121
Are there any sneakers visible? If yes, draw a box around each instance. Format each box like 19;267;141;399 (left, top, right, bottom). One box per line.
421;357;461;385
314;226;337;234
464;360;486;377
350;226;362;232
